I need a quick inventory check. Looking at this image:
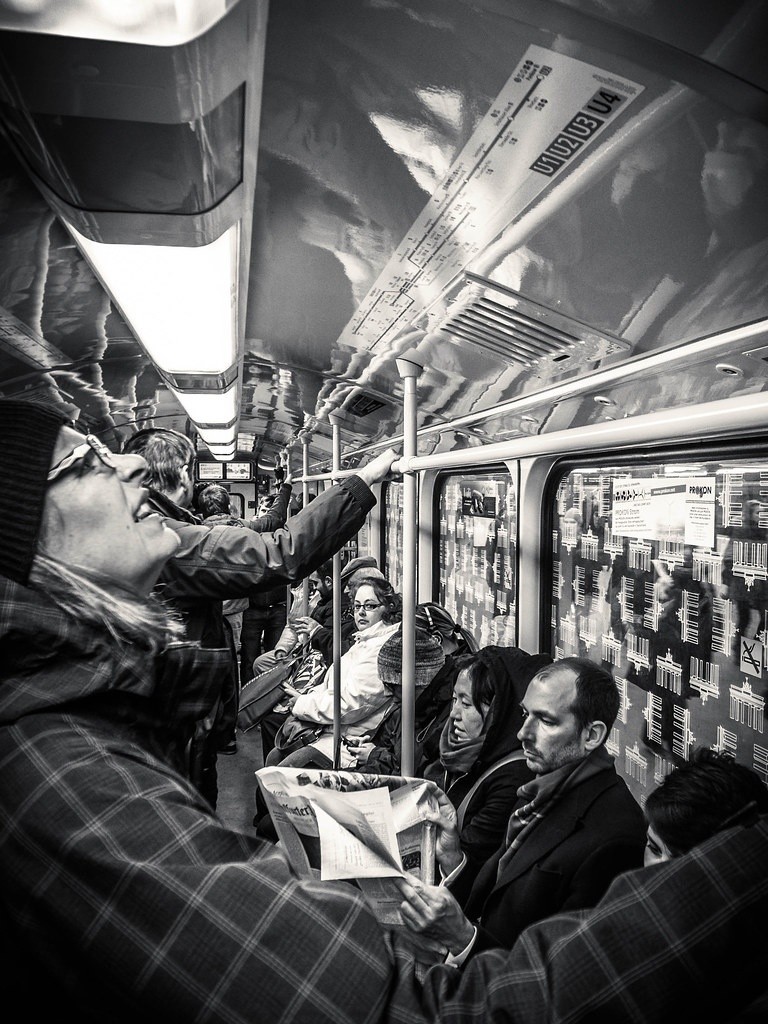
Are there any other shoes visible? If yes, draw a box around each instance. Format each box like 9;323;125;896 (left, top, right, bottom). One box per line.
217;741;236;754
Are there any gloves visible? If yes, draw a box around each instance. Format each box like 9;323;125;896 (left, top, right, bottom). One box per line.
274;467;284;479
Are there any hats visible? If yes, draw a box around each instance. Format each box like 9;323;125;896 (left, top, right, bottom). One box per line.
563;508;582;524
0;396;75;585
377;628;445;686
341;557;376;579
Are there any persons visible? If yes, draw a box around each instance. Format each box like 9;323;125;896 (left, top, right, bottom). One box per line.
0;398;768;1023
116;428;406;820
643;748;767;866
391;658;649;966
187;468;539;912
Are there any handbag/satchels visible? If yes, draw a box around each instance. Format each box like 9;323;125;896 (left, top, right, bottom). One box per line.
237;626;324;733
272;650;326;713
275;715;323;752
728;572;761;602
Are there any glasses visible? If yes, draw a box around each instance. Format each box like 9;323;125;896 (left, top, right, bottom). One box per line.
382;682;395;692
352;602;385;611
47;434;116;483
